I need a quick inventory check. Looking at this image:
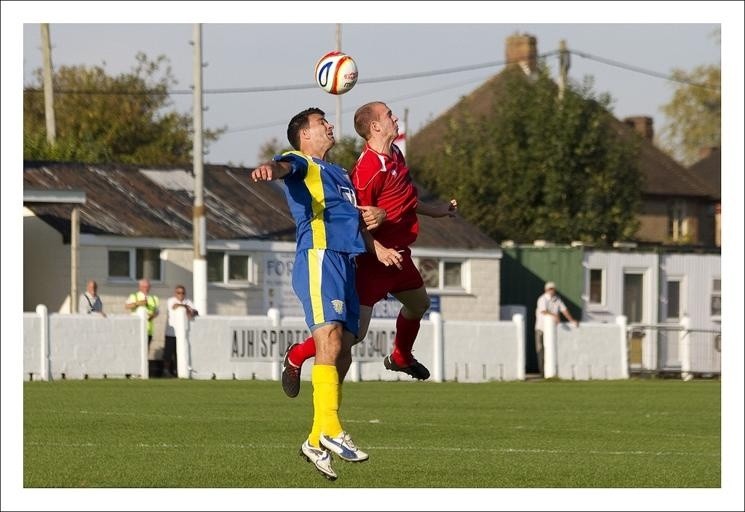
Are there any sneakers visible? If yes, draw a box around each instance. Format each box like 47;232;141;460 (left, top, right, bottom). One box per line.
297;438;338;481
281;343;302;398
319;429;369;463
383;352;431;381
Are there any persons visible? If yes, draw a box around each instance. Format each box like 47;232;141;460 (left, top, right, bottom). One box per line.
279;101;459;397
79;279;103;314
126;279;158;346
535;281;576;376
252;108;385;481
161;285;199;378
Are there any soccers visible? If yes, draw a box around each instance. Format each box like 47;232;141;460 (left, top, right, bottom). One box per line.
315;51;358;94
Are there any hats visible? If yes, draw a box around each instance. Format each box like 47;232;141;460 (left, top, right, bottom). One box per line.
544;280;556;291
